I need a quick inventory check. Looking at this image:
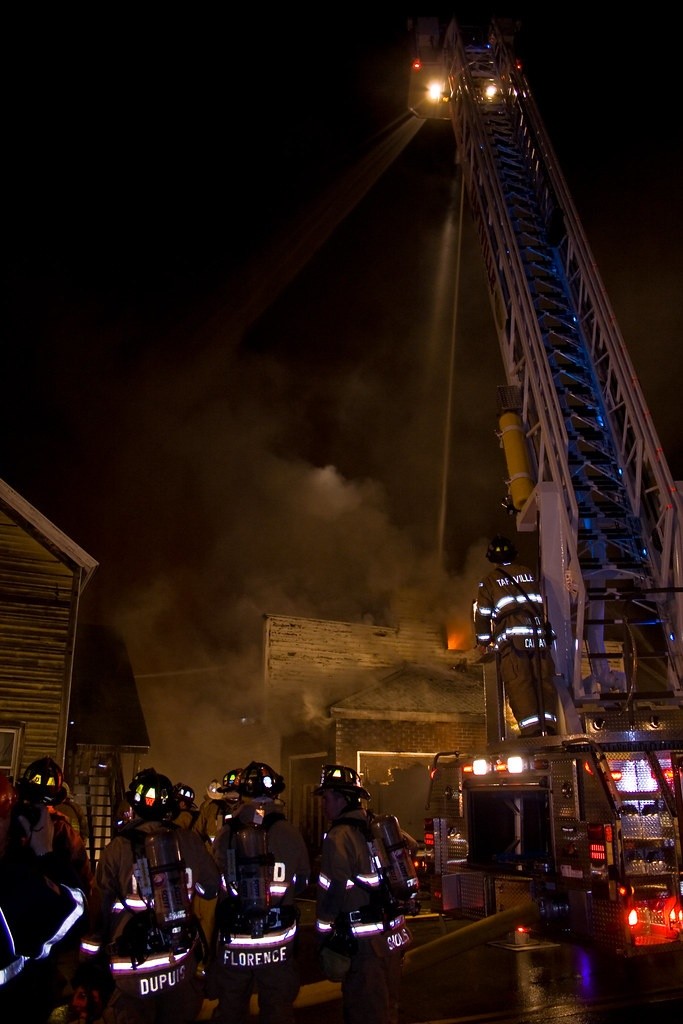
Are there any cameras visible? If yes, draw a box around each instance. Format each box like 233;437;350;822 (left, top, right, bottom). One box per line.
6;802;40;838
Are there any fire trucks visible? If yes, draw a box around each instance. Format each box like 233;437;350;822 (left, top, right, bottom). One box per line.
418;33;683;972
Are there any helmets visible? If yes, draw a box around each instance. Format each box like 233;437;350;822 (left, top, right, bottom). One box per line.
172;782;195;802
216;768;245;792
124;768;181;822
16;756;67;807
312;764;371;800
485;536;515;564
206;781;223;799
237;761;286;798
0;776;19;817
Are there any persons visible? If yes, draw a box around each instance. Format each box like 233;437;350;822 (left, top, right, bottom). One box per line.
474;536;557;738
0;759;418;1024
